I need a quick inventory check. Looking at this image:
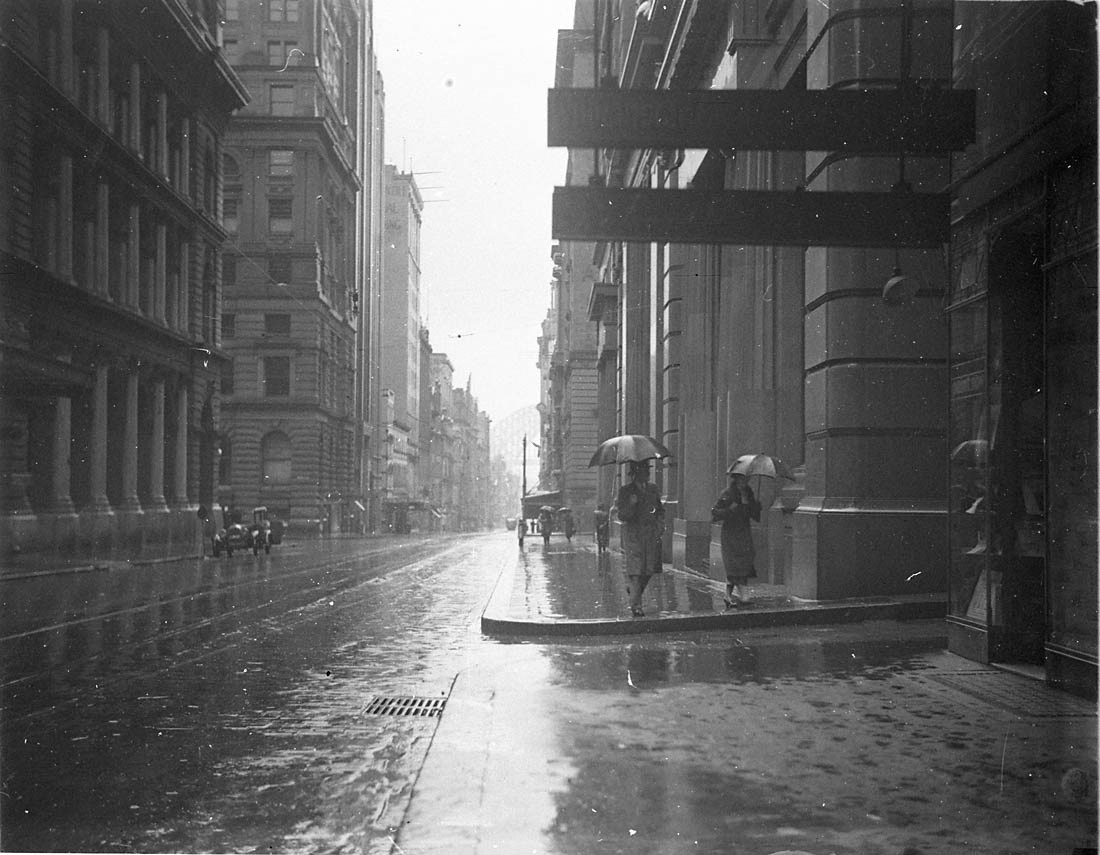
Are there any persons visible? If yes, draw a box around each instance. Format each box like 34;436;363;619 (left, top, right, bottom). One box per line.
618;460;666;617
562;511;573;542
594;503;609;553
712;474;762;606
539;511;553;543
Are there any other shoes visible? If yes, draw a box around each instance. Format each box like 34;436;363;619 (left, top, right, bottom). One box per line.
633;608;644;616
724;597;737;607
741;599;754;605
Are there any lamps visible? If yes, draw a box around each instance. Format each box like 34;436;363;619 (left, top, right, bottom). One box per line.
882;250;920;305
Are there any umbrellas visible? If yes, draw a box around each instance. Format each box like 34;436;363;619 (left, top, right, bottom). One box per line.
588;435;674;470
559;507;572;513
726;452;797;503
540;505;556;514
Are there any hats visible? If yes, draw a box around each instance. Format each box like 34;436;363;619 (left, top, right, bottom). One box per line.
628;460;653;475
